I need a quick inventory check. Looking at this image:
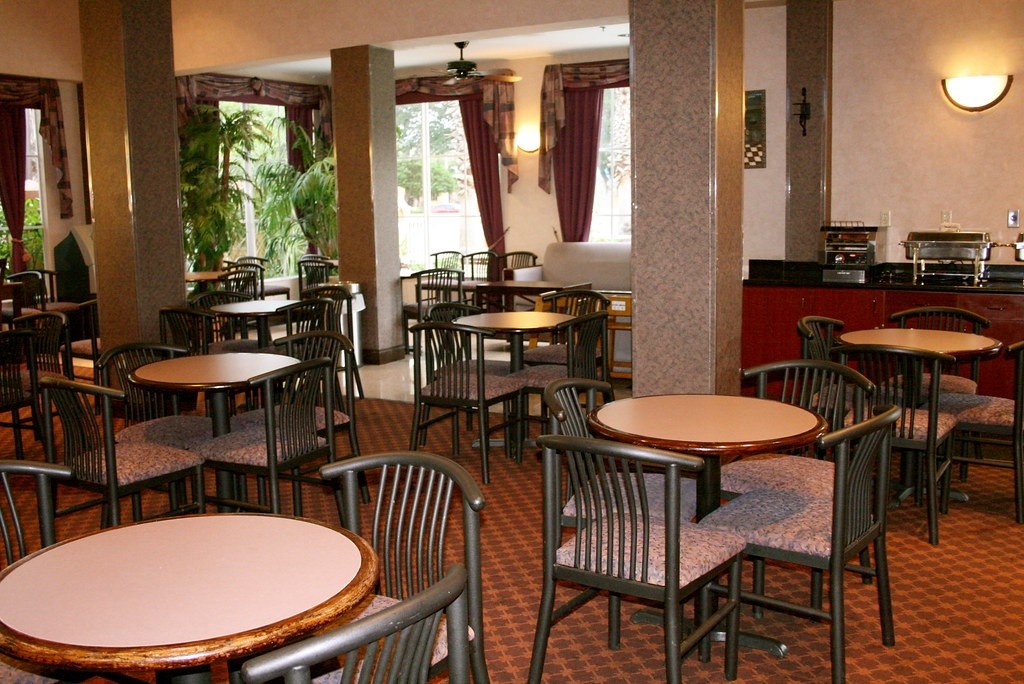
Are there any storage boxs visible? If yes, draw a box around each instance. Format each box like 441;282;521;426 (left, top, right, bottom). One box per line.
607;295;632;316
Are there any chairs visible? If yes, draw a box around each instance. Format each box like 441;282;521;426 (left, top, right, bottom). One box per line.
0;250;1024;684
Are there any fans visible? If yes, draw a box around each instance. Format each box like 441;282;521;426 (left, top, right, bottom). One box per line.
428;42;523;86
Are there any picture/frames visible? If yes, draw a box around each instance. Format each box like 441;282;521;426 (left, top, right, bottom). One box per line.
744;89;767;168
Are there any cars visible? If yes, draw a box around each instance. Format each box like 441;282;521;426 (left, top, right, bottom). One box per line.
432;204;459;214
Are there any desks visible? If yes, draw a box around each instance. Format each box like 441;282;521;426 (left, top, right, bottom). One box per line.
840;327;1003;509
474;281;592;314
450;310;576;456
129;352;302;512
0;512;377;684
211;300;305;349
586;394;829;657
186;271;228;283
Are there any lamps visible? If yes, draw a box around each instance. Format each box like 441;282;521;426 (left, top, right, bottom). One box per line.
940;75;1014;112
517;133;541;153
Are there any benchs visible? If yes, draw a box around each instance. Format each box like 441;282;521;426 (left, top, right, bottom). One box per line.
500;242;632;342
